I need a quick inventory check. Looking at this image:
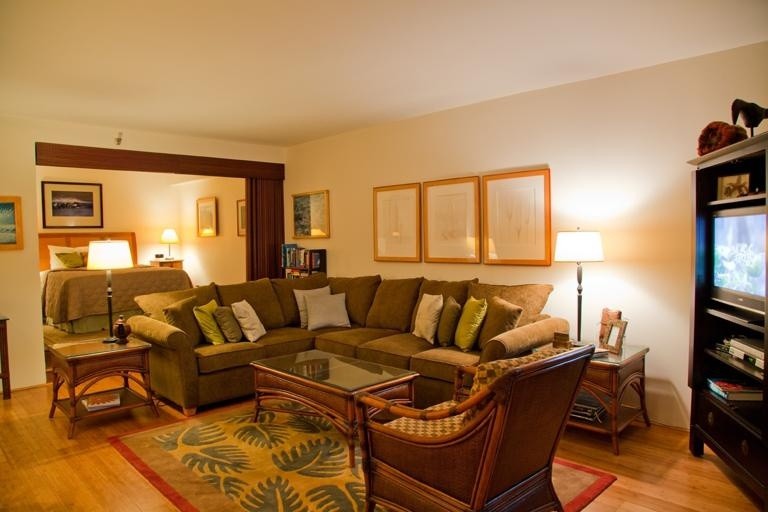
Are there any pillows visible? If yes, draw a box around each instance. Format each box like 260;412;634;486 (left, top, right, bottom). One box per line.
88;240;135;344
48;244;87;270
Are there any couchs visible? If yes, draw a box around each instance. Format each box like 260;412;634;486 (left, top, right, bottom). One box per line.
126;270;570;416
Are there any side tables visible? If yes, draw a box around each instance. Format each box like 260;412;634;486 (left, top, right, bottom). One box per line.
47;336;161;441
565;342;652;455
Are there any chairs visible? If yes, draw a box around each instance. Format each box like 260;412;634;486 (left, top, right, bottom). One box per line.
357;339;599;510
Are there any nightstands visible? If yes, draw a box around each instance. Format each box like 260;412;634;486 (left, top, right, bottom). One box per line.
150;258;183;270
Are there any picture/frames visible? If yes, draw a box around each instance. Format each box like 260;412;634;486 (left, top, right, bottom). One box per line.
372;168;550;266
602;319;631;354
236;199;248;236
194;195;217;238
290;189;331;240
42;181;104;229
0;195;25;253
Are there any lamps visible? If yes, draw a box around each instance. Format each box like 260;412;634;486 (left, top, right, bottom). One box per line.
158;228;180;260
555;227;605;348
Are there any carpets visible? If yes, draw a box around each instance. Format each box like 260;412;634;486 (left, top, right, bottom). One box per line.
111;358;616;512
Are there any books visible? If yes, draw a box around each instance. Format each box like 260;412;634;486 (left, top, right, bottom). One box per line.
570;394;608;422
706;375;763;402
593;347;608;358
282;244;320;279
82;391;121;412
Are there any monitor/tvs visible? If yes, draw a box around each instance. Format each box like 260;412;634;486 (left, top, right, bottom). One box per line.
708;205;768;325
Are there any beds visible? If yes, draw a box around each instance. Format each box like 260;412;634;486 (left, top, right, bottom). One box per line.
39;231;190;332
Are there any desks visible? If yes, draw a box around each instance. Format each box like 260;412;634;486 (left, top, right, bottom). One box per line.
0;317;11;401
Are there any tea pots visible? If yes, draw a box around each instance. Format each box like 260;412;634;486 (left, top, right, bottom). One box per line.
113;314;132;344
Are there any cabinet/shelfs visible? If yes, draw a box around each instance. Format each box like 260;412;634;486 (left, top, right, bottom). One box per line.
689;138;768;510
280;246;327;279
